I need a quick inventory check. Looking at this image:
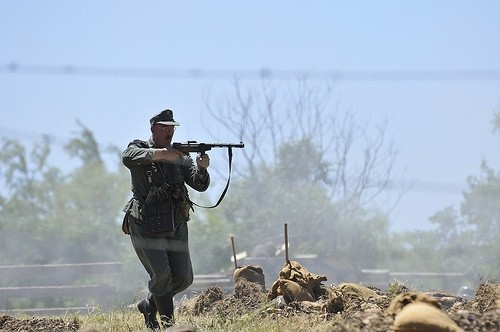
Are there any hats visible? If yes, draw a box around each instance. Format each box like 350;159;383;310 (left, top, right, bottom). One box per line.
149;108;181;126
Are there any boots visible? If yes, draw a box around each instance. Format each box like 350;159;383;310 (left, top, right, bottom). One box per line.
152;295;176;329
137;287;160;330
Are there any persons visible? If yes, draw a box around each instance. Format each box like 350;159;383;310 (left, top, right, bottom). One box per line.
120;108;211;332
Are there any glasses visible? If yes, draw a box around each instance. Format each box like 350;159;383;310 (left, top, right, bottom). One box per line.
154;127;176;131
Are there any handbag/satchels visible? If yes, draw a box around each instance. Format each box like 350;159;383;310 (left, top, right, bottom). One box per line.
144;199;175;233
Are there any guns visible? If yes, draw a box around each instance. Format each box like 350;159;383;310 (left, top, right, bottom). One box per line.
171;140;244;157
